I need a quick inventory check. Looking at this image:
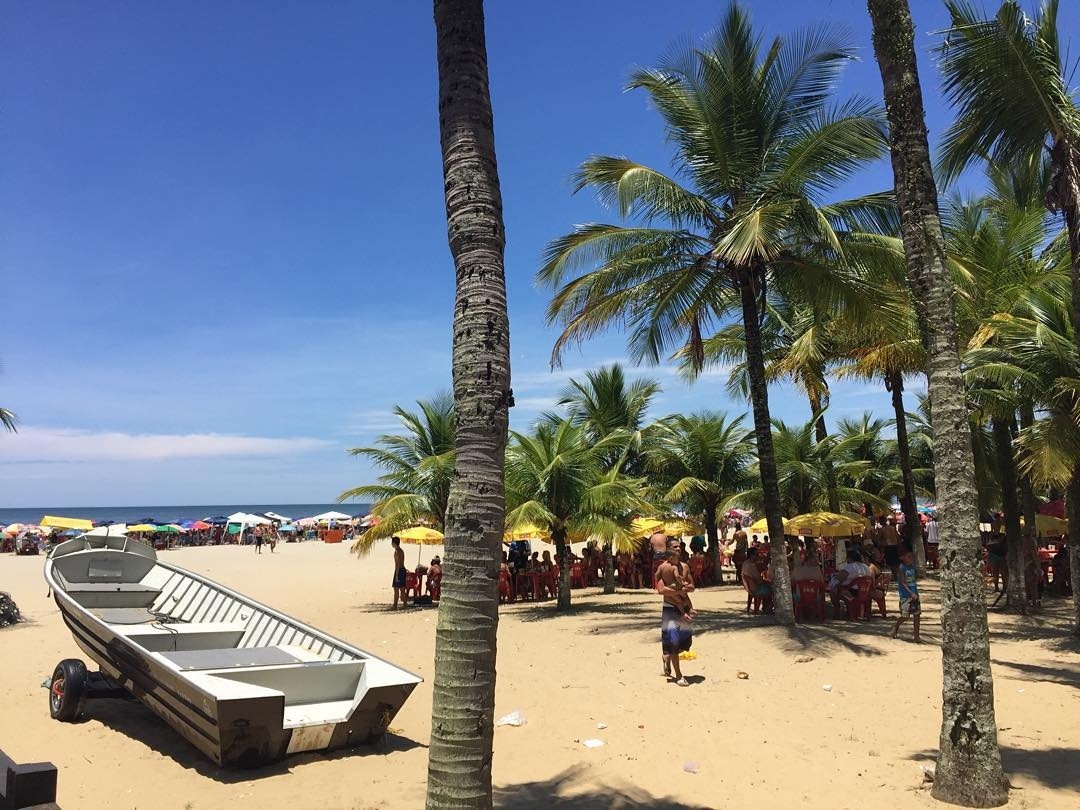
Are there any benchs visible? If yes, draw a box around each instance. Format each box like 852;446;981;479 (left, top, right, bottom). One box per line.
67;584;163;609
155;645;330;672
111;621;245;649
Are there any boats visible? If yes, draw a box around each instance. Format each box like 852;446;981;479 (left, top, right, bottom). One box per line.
43;525;427;770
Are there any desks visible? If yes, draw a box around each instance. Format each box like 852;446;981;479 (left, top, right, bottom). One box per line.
415;570;432;596
1039;549;1060;553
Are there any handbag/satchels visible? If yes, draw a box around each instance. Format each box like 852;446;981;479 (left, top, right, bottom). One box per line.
829;573;840;589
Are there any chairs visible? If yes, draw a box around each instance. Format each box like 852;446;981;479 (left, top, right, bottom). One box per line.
648;560;891;628
398;557;590;621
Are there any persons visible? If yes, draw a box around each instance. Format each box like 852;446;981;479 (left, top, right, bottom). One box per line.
498;510;1073;622
388;537;409;610
426;555;442;604
890;545;926;643
0;519;376;554
655;538;697;686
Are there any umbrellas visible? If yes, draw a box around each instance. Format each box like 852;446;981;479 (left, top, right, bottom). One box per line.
0;511;387;551
392;525;444;567
502;498;1069;575
